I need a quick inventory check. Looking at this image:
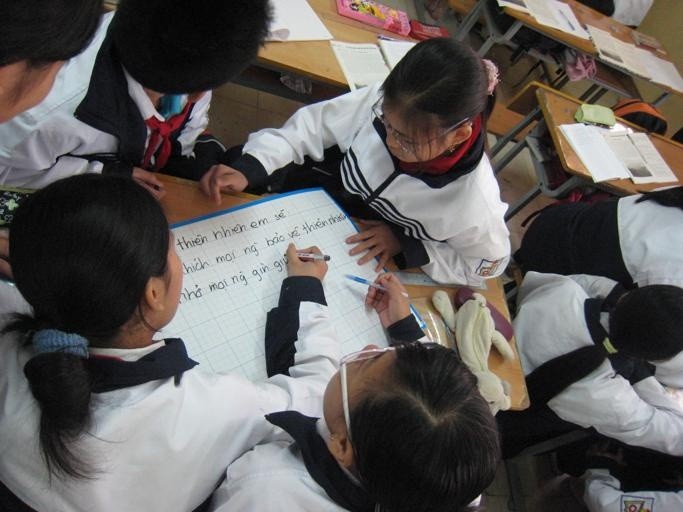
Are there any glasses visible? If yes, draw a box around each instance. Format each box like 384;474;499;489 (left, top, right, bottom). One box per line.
371;93;470;153
338;342;439;455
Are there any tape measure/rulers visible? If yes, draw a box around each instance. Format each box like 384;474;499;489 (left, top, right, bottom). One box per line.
393;272;487;290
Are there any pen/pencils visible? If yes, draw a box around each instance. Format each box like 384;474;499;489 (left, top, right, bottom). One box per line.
558;9;575;30
284;253;330;262
378;36;398;41
346;275;409;298
627;134;648;164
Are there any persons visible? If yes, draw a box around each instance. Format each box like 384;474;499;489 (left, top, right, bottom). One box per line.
196;35;513;285
494;259;683;461
571;454;683;512
1;174;339;511
205;274;502;512
0;0;108;282
0;1;274;205
505;187;683;290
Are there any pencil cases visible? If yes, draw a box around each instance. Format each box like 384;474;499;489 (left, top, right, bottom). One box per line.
336;0;411;36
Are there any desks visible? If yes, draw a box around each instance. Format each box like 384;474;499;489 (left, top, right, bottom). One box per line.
103;1;681;408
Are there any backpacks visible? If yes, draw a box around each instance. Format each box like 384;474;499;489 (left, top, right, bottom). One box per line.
612;97;667;134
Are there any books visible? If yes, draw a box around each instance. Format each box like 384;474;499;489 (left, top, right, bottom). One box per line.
557;124;680;185
261;0;333;42
329;38;418;90
497;0;683;91
